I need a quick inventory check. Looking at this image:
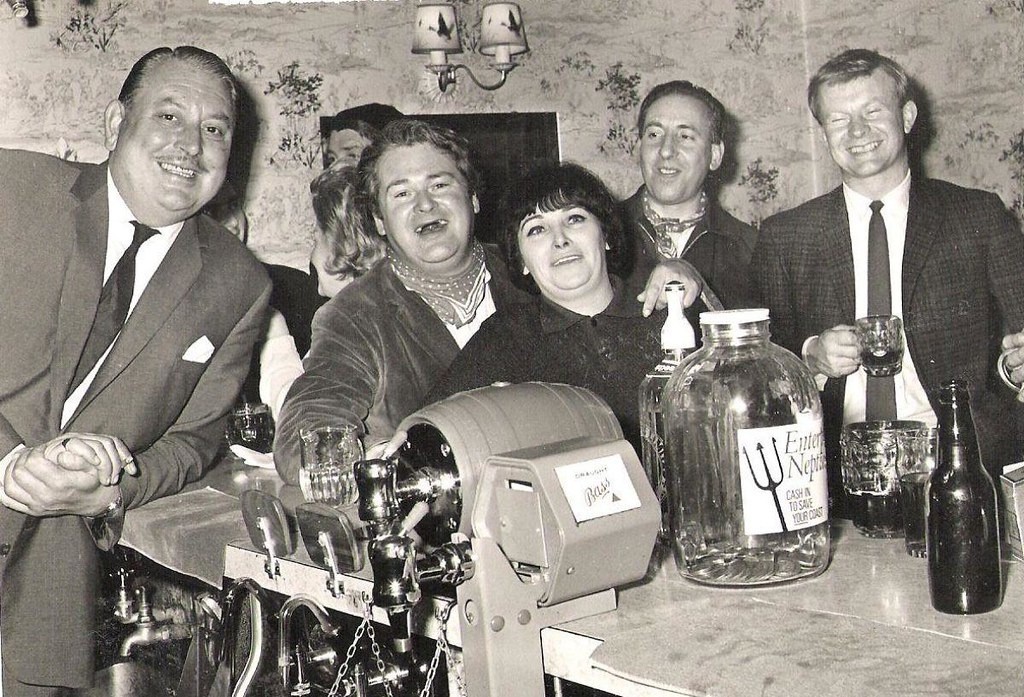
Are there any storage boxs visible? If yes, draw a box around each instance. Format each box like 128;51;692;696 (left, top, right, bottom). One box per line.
1000;461;1024;561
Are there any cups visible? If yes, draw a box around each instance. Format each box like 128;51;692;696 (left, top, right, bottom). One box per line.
851;316;905;376
225;404;275;454
297;425;364;508
896;428;941;558
840;421;927;539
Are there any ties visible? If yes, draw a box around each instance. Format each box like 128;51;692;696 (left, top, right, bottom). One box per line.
865;200;898;424
63;219;161;398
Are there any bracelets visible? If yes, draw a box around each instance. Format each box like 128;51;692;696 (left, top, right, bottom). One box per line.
99;493;122;513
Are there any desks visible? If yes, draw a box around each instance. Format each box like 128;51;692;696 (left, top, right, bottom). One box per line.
116;449;1024;697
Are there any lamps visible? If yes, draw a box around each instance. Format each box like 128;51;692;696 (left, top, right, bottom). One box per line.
412;3;526;103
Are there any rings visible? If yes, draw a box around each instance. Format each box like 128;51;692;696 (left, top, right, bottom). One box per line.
62;438;70;446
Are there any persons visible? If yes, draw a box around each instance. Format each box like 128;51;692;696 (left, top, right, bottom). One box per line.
328;104;404;161
615;81;759;310
417;163;710;453
199;180;310;404
745;48;1024;479
273;117;702;486
0;46;274;697
309;164;394;298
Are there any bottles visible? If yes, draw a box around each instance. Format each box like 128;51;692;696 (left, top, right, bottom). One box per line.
923;379;1003;614
639;280;698;549
661;308;829;586
